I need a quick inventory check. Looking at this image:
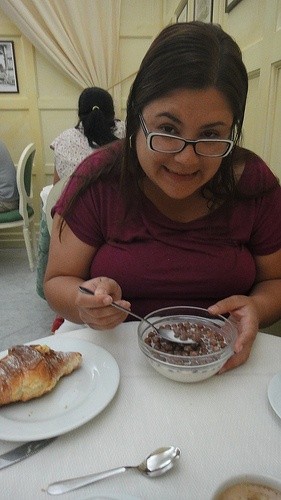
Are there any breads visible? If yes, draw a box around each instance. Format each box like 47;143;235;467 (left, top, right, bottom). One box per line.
0;344;82;406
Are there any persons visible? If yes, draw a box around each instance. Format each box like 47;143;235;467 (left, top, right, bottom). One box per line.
44;21;281;376
0;141;19;213
51;87;126;332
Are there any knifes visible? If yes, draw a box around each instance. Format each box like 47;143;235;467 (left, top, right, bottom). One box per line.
0;436;57;470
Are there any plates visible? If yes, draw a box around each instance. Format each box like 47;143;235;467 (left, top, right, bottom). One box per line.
267;371;281;418
0;336;121;444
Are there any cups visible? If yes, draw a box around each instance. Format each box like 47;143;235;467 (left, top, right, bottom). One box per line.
212;471;281;500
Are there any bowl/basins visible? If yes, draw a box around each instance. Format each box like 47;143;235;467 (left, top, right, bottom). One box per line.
137;306;237;383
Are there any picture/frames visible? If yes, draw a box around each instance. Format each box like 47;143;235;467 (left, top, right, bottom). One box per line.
176;0;239;24
0;41;20;93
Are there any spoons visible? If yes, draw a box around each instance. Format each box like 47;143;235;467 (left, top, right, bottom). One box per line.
79;286;200;345
46;444;182;496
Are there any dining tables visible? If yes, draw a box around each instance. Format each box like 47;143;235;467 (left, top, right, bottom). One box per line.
0;321;281;500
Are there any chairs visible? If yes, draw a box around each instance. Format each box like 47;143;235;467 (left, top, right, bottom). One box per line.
0;143;37;273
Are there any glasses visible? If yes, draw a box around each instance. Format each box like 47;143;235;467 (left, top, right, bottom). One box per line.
139;108;234;157
